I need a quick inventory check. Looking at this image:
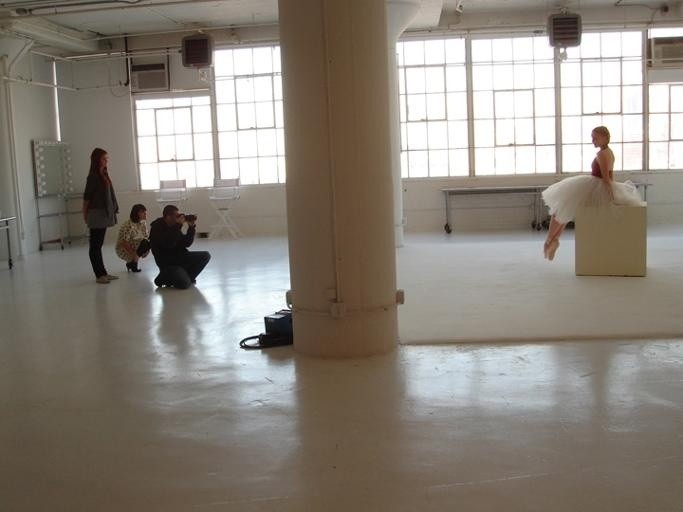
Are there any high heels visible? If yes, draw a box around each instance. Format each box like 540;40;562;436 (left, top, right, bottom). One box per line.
126;262;141;272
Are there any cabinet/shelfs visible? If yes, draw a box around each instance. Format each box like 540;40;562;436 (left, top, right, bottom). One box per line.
36;193;89;251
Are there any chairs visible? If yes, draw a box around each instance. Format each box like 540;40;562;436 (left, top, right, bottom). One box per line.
208;178;240;240
157;180;188;213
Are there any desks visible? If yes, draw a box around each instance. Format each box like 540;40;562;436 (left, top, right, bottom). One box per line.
1;216;15;270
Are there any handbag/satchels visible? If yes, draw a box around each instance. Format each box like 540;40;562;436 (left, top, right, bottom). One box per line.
240;332;293;349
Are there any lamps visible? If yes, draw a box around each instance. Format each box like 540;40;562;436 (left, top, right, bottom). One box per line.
32;140;73;198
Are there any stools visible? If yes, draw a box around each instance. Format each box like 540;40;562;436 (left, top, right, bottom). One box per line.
576;202;647;276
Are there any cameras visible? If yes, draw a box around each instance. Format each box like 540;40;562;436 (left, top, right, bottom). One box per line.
177;213;197;222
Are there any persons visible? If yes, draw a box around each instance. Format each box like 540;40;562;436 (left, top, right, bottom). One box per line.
116;204;150;272
541;126;642;260
82;147;120;283
150;205;211;290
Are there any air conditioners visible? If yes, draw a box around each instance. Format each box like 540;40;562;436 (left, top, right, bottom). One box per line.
131;62;169;93
648;37;683;67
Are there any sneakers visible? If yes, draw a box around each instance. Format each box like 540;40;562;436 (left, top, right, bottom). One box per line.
544;241;559;260
108;275;119;280
96;277;110;283
